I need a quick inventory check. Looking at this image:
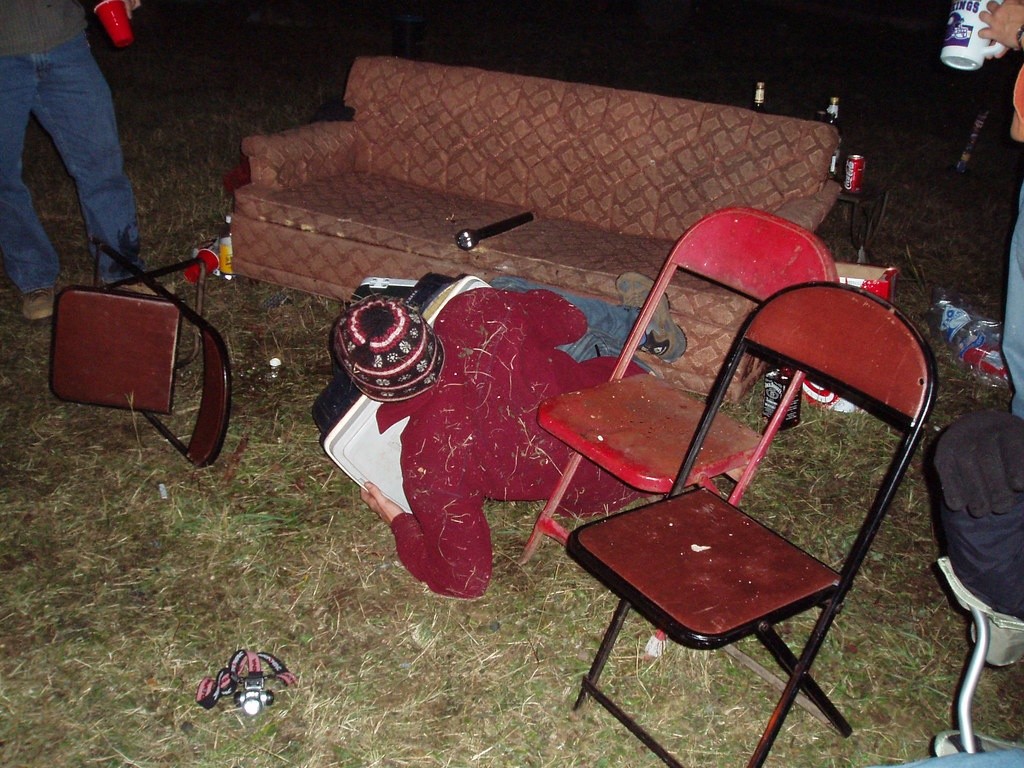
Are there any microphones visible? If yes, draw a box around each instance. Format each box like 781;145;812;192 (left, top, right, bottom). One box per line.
456;211;535;251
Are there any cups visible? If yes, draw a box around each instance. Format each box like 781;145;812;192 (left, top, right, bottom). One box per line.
94;0;134;48
182;248;220;283
940;0;1004;72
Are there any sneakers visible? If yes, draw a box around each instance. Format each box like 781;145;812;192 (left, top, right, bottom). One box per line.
95;274;158;300
22;286;51;322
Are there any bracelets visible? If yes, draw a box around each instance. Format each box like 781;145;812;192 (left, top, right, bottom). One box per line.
1017;24;1024;51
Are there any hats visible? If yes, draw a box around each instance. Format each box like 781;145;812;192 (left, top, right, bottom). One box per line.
331;294;446;403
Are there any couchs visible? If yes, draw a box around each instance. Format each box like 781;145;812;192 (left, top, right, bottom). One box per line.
231;58;844;404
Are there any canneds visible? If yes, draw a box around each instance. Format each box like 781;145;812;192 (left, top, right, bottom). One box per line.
845;154;865;192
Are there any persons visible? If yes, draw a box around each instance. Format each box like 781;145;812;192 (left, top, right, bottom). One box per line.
930;0;1024;666
0;0;176;322
334;272;690;598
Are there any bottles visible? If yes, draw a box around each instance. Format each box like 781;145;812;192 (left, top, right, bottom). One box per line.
826;97;843;178
751;82;768;114
218;216;235;275
762;363;802;430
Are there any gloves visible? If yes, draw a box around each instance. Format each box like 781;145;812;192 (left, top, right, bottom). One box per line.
934;409;1024;518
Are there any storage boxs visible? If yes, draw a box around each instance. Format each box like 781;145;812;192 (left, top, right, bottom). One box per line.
782;263;897;414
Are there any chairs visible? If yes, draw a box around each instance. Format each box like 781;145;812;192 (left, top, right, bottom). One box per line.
564;280;939;768
48;235;232;468
515;206;841;656
